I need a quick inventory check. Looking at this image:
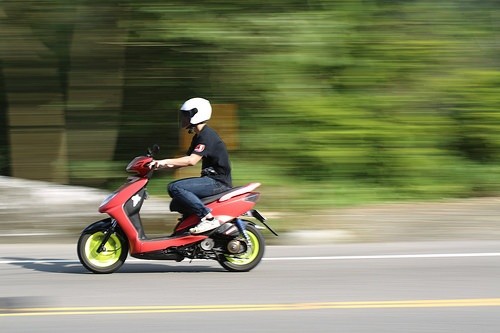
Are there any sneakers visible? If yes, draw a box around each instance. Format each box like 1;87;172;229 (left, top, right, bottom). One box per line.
189;216;220;234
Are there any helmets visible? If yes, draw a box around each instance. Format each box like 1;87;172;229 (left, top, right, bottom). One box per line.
179;98;212;129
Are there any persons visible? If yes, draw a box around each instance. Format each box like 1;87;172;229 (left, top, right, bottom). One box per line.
148;97;233;236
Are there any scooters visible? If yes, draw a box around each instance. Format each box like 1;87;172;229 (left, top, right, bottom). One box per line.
76;143;279;274
